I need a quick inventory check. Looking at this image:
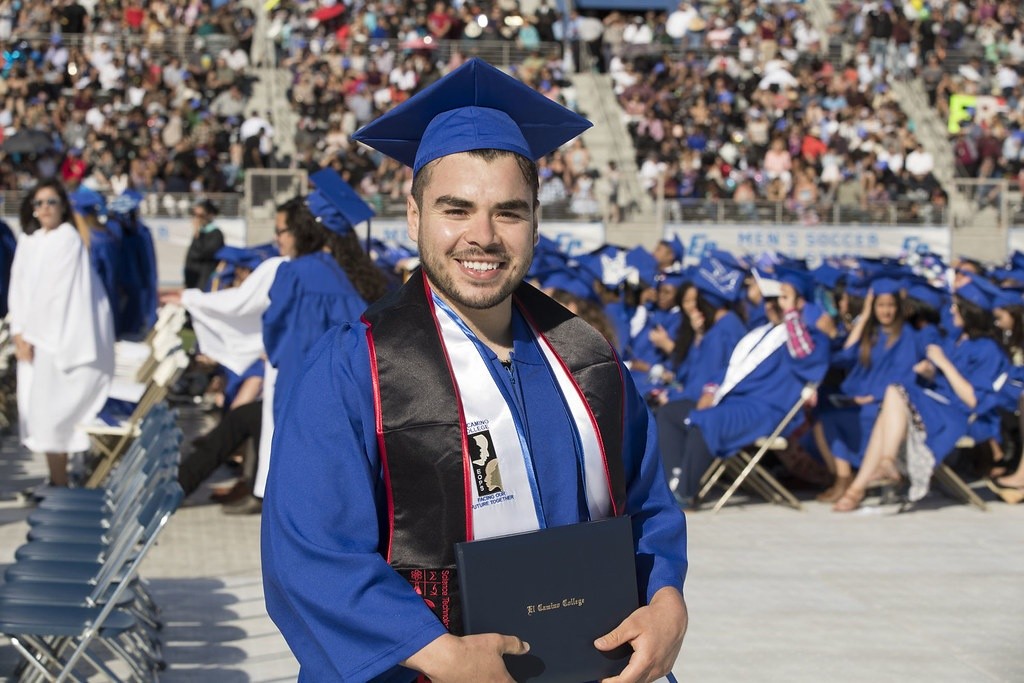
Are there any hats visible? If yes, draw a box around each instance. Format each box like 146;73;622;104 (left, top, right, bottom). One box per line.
303;167;377;237
206;234;1024;312
66;184;109;216
351;56;595;178
112;187;143;214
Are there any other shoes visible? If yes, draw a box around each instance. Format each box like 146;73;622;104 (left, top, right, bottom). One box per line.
897;491;936;514
223;492;262;515
671;490;700;512
879;456;905;485
834;485;867;512
209;480;255;503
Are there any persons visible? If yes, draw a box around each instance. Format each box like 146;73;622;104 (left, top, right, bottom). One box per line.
1;1;1023;519
260;54;690;683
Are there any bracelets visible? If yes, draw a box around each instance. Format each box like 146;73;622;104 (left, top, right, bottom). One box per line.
702;383;718;394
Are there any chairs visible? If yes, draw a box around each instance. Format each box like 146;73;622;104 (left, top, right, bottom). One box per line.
916;404;990;512
693;378;823;512
0;301;191;683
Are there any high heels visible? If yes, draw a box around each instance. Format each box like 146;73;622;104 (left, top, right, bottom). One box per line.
984;473;1024;505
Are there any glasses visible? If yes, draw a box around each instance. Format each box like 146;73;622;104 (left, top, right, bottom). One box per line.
30;198;61;206
190;211;206;219
274;227;290;236
